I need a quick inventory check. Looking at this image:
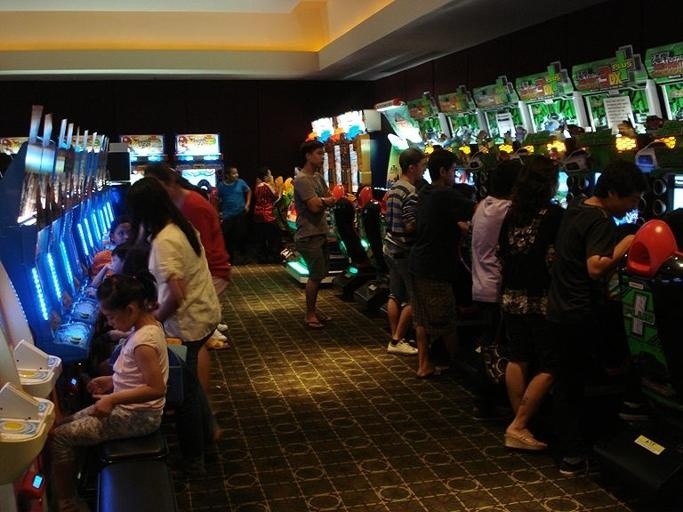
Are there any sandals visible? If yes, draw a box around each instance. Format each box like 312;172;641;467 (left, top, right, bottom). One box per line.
206;339;230;349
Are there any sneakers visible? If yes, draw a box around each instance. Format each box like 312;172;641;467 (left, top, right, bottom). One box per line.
211;323;228;342
559;451;605;475
619;402;648;421
387;338;418;354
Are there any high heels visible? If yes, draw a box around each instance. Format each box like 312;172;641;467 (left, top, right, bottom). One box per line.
504;429;548;450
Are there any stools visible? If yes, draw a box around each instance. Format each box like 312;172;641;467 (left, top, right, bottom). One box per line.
91;455;178;511
100;432;168;463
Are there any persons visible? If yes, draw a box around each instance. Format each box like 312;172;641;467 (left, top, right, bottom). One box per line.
294;140;335;328
91;165;232;473
47;270;170;509
252;166;281;264
381;147;647;477
217;167;252;264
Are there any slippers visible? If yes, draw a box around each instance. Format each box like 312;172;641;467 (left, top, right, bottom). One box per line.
417;366;449;379
305;307;332;328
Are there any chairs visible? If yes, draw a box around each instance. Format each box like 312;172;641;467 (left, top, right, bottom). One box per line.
327;195;378;303
359;198;409;318
